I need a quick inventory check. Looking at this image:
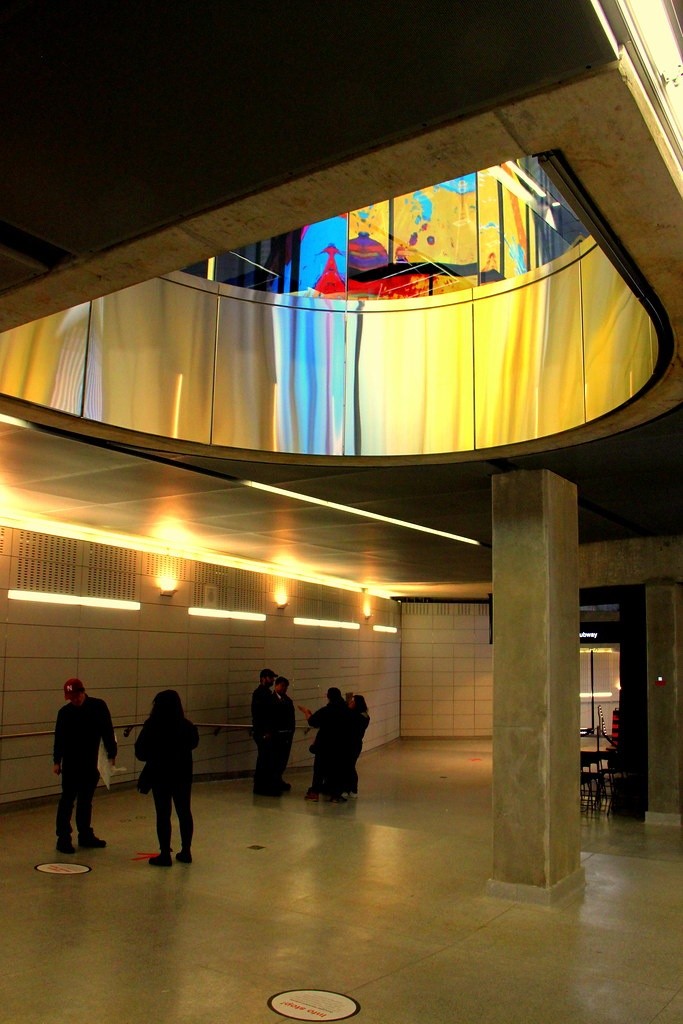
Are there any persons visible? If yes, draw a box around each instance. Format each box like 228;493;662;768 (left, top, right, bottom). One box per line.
53;678;117;854
299;687;370;802
134;689;200;867
251;669;296;798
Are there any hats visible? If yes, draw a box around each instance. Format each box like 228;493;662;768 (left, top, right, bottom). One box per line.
64;678;82;700
260;669;278;678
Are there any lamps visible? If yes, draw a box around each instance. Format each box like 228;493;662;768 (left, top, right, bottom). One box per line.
277;603;291;609
365;615;373;619
160;590;179;597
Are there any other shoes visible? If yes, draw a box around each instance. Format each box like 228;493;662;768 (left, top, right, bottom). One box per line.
176;852;192;863
279;779;291;792
253;783;283;797
56;836;74;853
303;791;358;802
149;853;172;867
76;828;106;848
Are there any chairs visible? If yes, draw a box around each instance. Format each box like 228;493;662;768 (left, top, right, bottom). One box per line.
580;750;628;815
